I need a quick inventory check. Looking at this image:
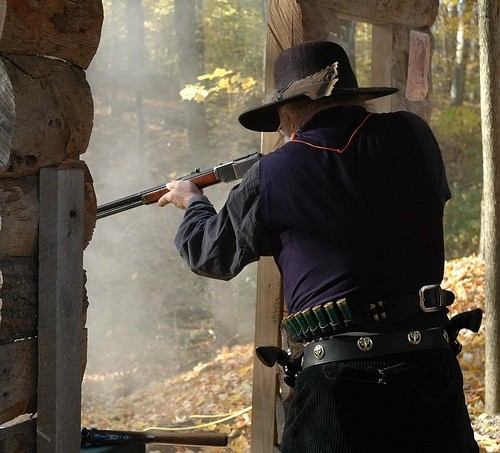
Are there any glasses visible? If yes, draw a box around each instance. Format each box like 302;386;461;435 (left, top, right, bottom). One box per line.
277;113;287;137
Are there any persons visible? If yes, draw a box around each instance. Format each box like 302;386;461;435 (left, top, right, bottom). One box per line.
156;39;489;453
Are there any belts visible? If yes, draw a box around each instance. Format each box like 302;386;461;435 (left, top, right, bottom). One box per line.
300;327;455;370
351;283;455;329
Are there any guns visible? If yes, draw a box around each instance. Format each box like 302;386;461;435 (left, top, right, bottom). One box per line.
94;148;263;219
79;426;230;449
448;308;483;358
255;344;301;390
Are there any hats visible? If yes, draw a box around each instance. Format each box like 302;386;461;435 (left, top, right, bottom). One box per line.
238;40;400;133
